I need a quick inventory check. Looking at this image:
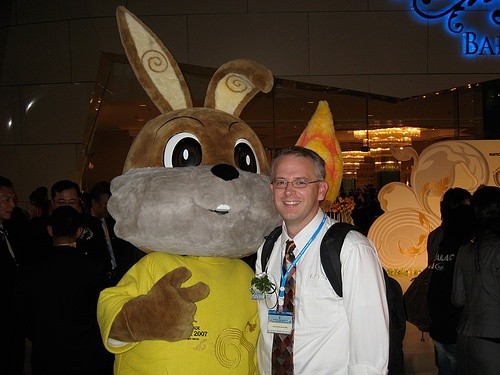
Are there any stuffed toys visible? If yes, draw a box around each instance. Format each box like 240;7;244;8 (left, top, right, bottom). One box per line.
97;6;284;375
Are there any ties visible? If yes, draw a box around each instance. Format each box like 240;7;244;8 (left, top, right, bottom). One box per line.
272;239;296;375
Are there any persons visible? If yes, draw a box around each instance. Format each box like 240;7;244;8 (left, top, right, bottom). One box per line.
327;184;500;375
0;176;146;375
255;146;389;375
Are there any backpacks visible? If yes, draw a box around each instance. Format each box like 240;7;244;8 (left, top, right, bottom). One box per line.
261;222;407;375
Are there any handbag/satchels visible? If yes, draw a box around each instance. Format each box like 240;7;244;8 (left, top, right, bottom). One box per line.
403;262;458;345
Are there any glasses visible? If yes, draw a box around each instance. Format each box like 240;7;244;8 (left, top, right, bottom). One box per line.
271;177;322;189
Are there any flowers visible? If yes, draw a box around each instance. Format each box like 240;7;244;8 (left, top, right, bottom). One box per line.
249;275;275;300
79;228;94;241
